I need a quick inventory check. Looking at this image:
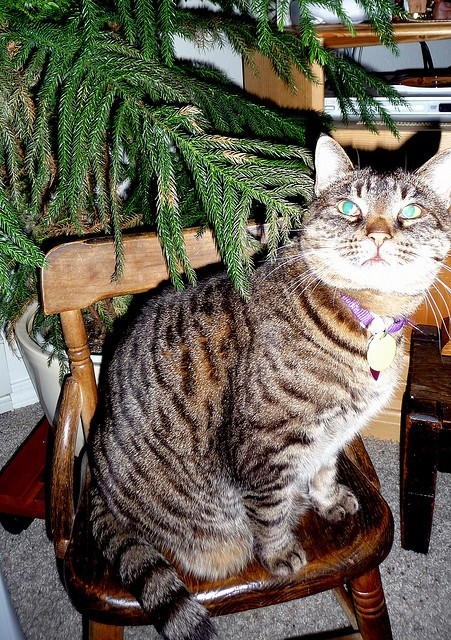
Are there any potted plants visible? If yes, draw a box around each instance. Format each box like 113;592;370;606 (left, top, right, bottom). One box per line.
0;2;427;462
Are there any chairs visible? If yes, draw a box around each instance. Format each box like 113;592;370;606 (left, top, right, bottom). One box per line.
38;219;393;638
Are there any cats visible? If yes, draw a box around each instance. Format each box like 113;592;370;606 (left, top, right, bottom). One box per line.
83;133;449;633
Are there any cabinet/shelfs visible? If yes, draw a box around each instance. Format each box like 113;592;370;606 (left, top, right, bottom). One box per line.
241;17;450;436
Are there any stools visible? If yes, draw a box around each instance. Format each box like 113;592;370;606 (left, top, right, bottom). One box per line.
400;318;450;553
